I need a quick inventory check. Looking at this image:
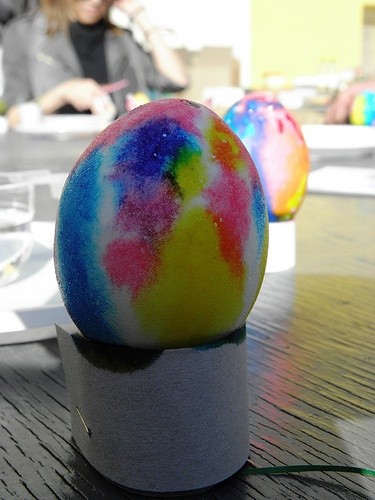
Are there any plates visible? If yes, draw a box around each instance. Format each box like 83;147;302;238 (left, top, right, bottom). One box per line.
300;124;375;159
13;113;112;141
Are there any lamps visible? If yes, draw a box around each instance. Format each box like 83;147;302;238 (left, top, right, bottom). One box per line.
52;97;268;494
349;91;375;127
222;90;312;276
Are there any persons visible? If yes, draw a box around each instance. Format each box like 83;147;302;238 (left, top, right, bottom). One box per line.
1;0;190;119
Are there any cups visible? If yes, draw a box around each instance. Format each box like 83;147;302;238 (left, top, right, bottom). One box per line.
0;171;36;283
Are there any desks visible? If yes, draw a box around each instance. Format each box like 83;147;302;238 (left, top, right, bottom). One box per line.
1;104;374;499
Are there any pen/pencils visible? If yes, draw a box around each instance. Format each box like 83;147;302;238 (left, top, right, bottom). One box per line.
102;79;130;93
